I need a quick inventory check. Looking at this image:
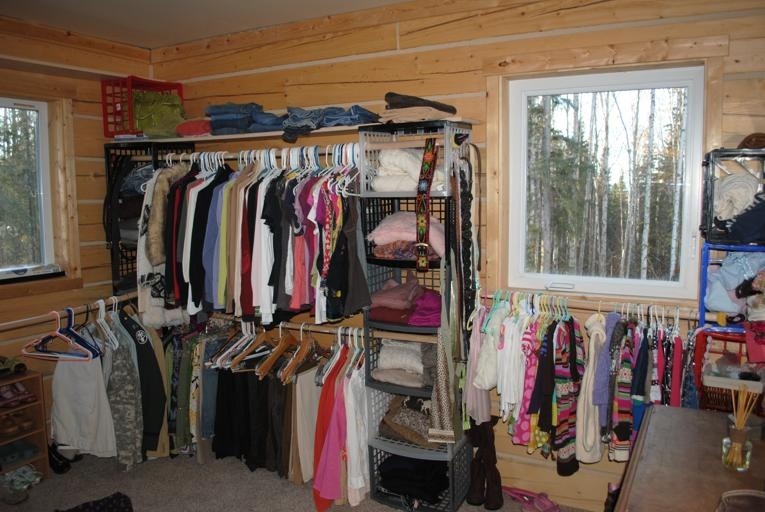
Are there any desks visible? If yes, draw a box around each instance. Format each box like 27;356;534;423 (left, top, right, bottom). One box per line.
605;398;765;511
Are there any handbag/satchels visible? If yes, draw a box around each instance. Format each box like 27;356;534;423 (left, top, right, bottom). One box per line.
121;91;184;138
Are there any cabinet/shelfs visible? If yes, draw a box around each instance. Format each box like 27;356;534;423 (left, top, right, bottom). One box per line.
696;146;764;420
95;111;487;511
0;364;52;488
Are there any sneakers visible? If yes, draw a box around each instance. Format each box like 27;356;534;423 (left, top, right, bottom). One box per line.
0;355;45;505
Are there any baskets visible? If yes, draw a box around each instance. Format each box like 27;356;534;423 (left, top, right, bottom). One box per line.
702;366;762;414
101;75;184;138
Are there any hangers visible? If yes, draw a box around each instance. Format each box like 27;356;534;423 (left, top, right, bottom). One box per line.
22;293;131;366
134;138;391;200
202;315;375;385
463;286;698;349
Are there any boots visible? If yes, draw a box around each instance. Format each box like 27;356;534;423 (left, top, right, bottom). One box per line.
466;415;503;510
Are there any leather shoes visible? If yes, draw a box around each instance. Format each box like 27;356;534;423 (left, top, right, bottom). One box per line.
48;444;83;474
